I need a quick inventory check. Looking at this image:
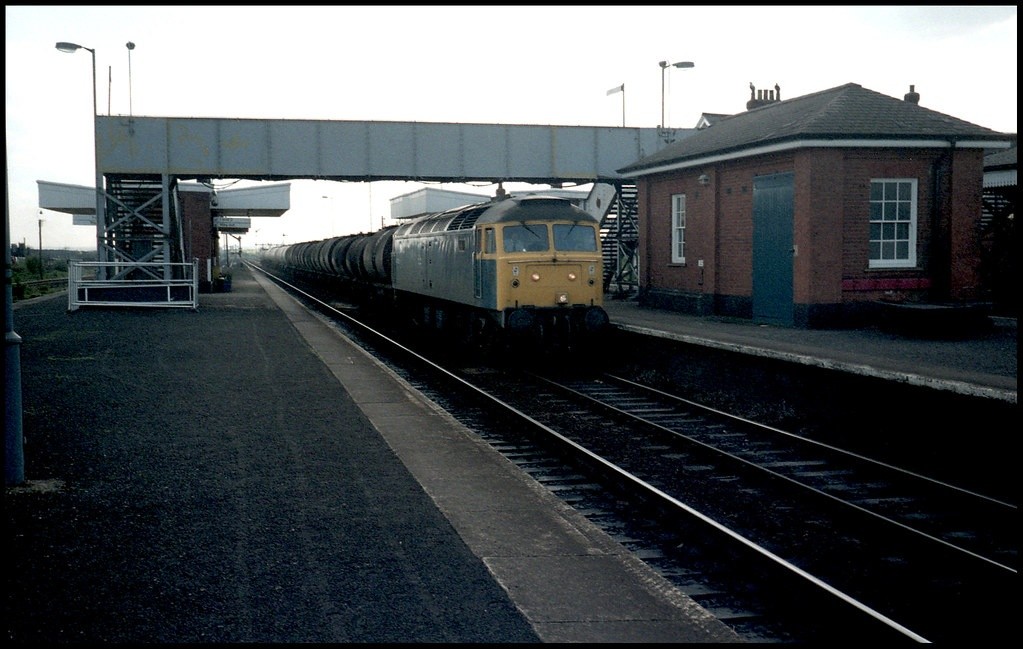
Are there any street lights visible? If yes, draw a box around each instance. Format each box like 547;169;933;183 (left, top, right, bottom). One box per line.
53;41;98;116
659;59;695;128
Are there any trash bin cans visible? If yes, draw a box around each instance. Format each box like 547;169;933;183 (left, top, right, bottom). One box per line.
215;273;231;292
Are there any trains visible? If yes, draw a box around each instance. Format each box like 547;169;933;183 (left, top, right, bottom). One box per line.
255;184;605;330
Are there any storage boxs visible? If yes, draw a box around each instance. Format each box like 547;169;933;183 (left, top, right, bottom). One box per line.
213;280;232;292
